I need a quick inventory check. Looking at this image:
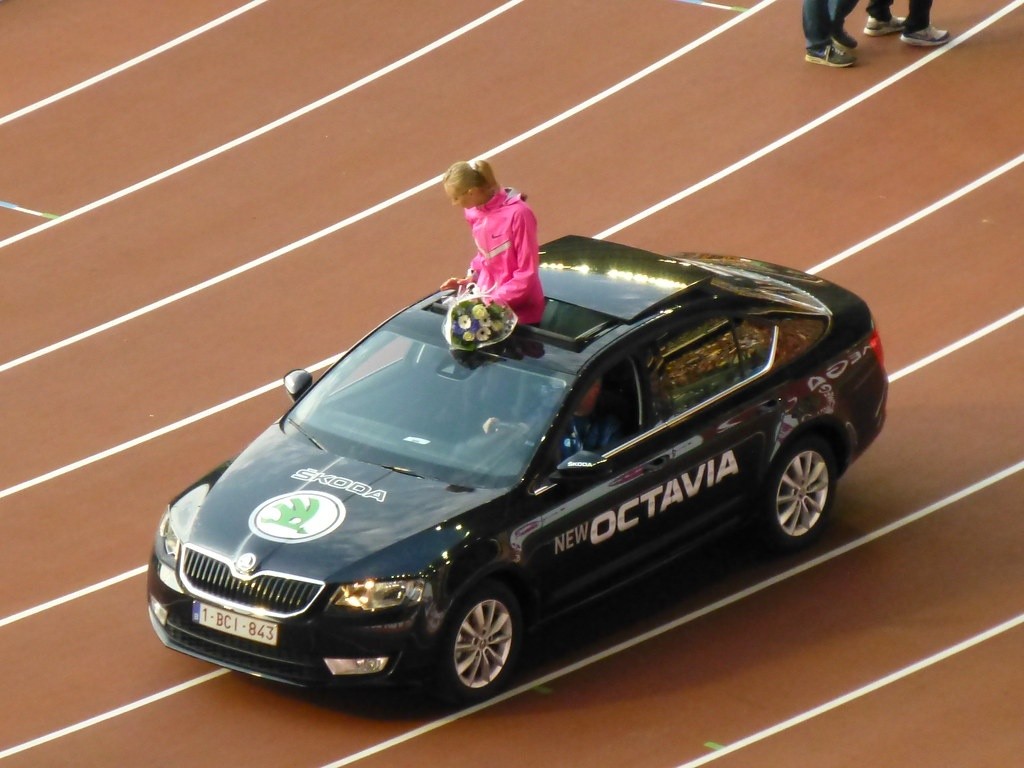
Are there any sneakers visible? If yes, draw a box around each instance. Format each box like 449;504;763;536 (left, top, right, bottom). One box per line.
863;16;906;36
805;41;856;68
900;25;950;45
830;31;858;50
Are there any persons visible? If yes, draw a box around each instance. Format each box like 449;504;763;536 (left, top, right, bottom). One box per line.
483;374;624;462
863;0;951;46
802;0;859;68
440;160;546;328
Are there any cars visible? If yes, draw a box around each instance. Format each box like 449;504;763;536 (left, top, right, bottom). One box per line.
148;233;890;719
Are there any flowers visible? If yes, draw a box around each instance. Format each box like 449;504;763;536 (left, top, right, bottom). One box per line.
441;282;518;351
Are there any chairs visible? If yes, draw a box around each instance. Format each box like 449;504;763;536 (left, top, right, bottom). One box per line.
597;368;639;437
